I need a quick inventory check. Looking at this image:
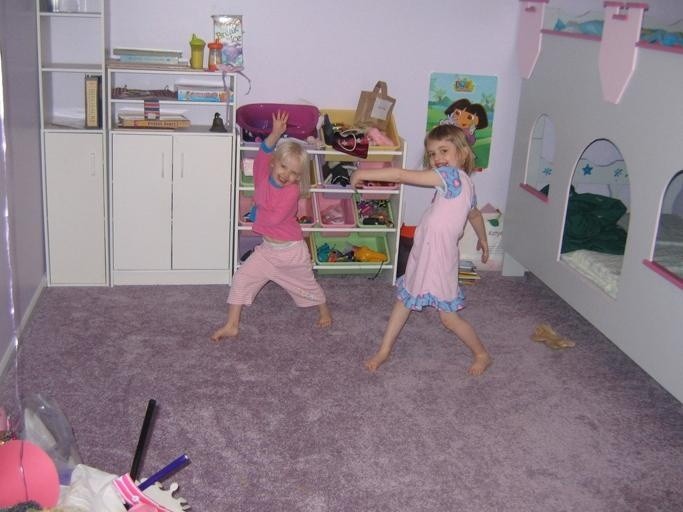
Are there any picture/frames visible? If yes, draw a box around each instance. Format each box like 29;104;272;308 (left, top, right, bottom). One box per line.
518;112;556;205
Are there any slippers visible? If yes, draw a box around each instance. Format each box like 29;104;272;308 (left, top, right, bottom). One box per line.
544;335;575;348
528;323;562;341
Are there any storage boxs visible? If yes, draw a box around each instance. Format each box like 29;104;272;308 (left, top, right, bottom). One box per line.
317;150;353;199
317;108;400;163
354;158;399;202
240;169;255;196
238;233;263;262
311;224;390;277
352;193;393;237
314;184;356;237
238;194;316;237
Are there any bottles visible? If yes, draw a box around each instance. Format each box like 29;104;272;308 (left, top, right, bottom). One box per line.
207;38;223;70
189;34;206;69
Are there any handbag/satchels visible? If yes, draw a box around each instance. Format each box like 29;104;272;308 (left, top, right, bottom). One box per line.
350;80;397;130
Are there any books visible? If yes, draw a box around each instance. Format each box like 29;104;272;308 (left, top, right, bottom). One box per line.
458;260;481;285
85;73;102;129
113;48;182;63
176;85;234;101
118;114;191;127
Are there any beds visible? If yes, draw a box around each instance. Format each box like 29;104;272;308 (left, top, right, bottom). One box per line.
556;158;683;299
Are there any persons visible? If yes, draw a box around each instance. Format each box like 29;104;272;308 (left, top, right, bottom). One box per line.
211;109;331;341
350;124;492;376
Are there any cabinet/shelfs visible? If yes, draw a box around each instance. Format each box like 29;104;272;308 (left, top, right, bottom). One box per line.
232;108;409;286
42;132;108;285
36;1;106;130
108;68;234;285
